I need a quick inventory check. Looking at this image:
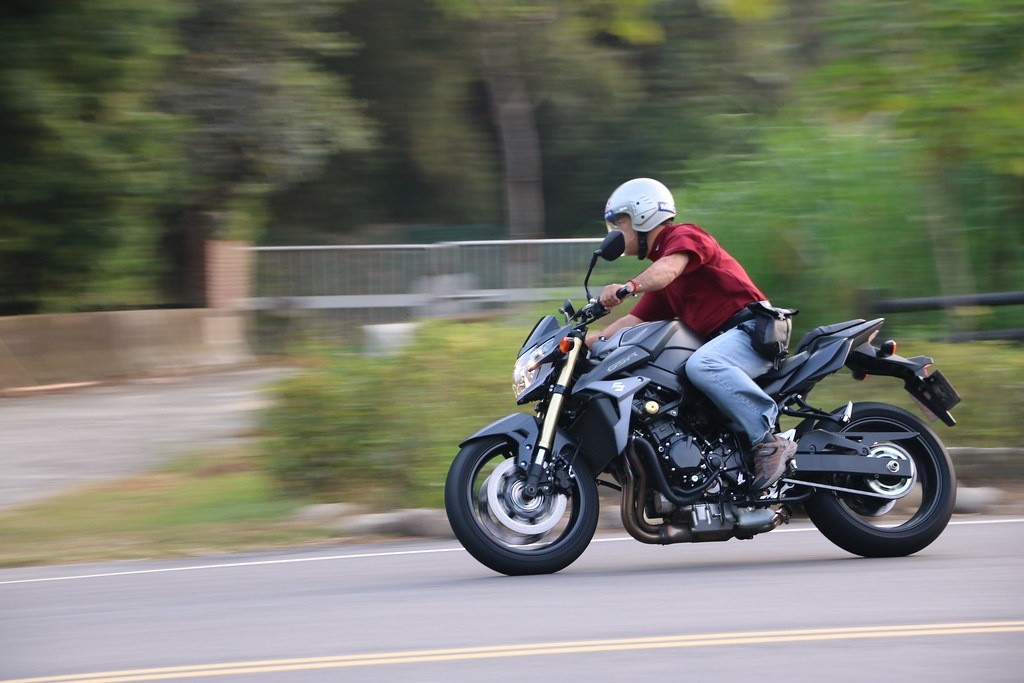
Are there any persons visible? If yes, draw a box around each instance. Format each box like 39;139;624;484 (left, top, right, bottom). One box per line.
587;177;798;490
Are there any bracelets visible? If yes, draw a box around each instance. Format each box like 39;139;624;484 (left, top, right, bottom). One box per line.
628;279;638;297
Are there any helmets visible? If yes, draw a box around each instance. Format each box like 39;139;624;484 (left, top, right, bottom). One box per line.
604;178;676;232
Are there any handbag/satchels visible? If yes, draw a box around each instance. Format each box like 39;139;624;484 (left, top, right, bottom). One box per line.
749;300;800;361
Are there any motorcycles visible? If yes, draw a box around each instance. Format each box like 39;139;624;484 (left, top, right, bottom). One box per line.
443;228;961;577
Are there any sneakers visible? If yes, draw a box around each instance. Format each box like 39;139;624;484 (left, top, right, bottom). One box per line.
751;436;800;491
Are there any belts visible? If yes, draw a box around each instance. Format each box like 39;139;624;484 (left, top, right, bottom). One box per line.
707;308;754;343
